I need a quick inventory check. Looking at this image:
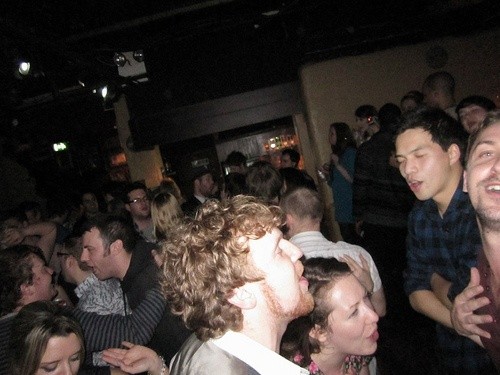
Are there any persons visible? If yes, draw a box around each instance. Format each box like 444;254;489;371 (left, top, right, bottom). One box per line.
0;300;175;375
346;67;497;160
278;257;378;375
391;104;476;332
79;209;195;364
57;229;136;317
352;101;422;338
451;112;499;375
0;144;319;263
318;122;364;251
0;243;170;375
155;193;317;374
276;185;386;319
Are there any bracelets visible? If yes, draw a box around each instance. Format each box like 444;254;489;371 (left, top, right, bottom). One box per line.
147;354;167;375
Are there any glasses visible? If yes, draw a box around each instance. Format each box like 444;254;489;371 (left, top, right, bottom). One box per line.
128;196;149;202
